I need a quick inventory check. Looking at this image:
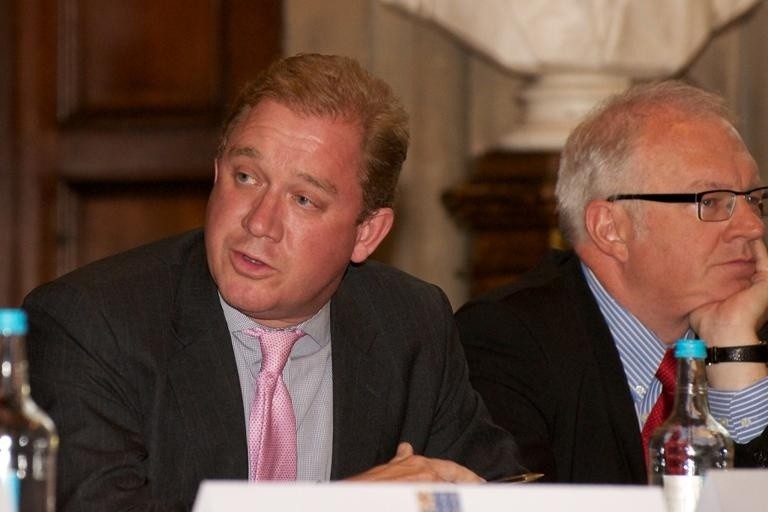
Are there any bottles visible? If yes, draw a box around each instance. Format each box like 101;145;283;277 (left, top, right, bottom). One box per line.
0;305;63;510
650;338;735;512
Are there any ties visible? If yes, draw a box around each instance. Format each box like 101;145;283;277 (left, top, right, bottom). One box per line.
238;324;307;486
641;350;690;489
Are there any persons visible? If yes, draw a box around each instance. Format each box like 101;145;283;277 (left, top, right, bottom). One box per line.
454;76;767;489
0;50;540;512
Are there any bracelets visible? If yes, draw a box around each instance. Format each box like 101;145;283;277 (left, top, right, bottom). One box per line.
705;343;767;367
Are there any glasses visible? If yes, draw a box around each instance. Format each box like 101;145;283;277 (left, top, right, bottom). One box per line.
605;183;768;224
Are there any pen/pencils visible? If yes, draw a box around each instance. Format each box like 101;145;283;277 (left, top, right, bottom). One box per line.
495;472;544;483
